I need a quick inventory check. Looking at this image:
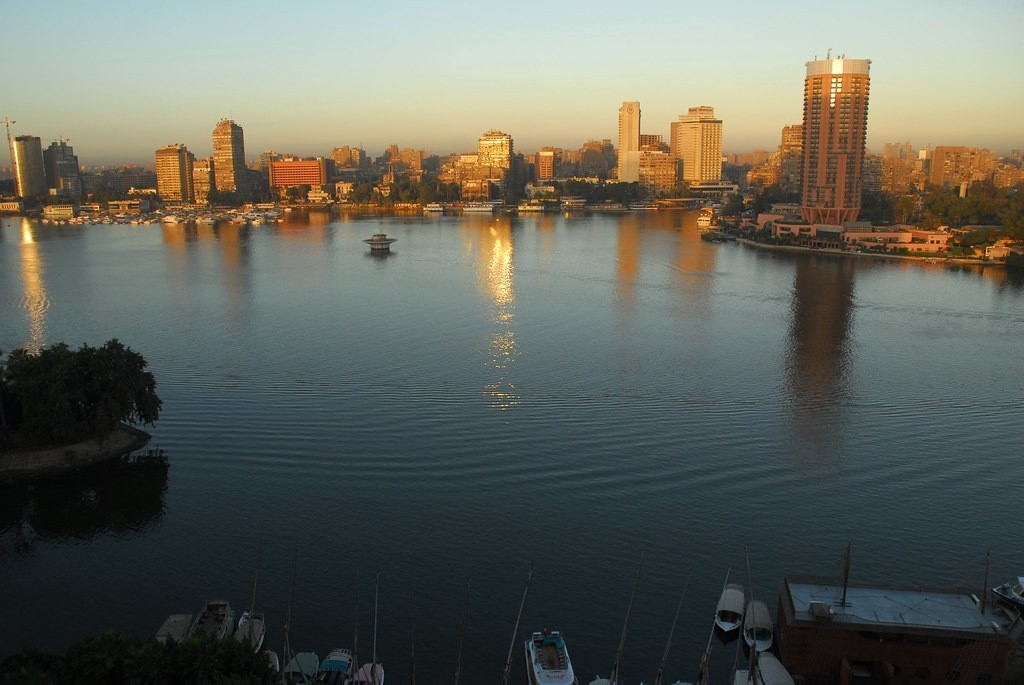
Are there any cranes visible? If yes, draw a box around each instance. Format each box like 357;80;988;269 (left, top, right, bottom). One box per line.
42;134;70;144
0;115;18;196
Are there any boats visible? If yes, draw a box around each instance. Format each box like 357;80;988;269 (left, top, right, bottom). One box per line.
697;215;710;227
991;577;1024;607
30;205;285;225
264;652;279;673
924;260;936;264
189;602;236;643
712;203;722;213
153;613;192;644
714;582;745;632
422;202;448;212
755;652;795;685
524;630;578;685
628;200;659;210
733;670;763;685
234;608;266;655
743;599;775;652
317;647;354;685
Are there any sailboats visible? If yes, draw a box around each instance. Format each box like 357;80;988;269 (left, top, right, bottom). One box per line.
462;182;499;212
589;549;645;685
353;573;385;685
278;620;319;685
